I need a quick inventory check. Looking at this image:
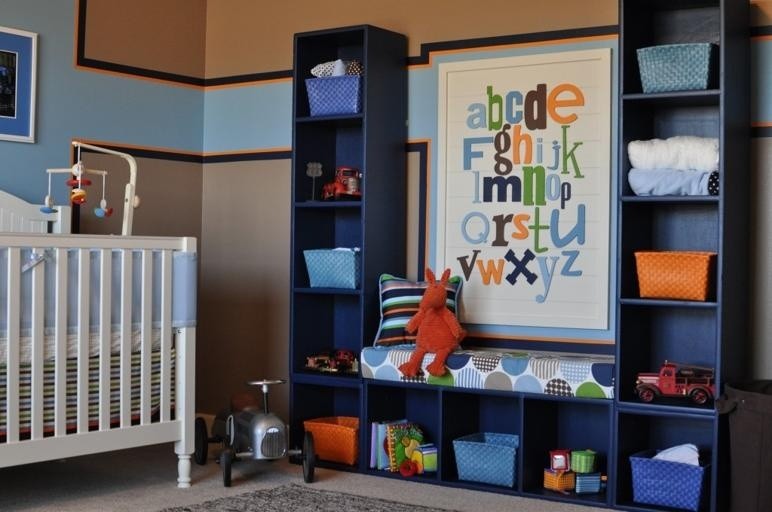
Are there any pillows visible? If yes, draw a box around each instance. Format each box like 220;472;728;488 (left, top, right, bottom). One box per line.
374;273;463;351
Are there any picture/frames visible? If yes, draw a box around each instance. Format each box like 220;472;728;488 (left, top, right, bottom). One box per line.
0;25;39;144
434;46;614;334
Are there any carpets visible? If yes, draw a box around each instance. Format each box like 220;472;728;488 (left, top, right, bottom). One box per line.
147;483;460;512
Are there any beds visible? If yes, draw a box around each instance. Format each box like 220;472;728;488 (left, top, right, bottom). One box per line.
0;190;199;489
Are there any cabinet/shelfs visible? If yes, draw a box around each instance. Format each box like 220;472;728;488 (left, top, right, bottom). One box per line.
288;0;754;512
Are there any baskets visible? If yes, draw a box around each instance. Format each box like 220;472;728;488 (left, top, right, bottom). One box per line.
634;251;717;302
303;249;362;289
303;416;359;465
305;75;362;117
453;432;519;488
637;42;718;93
629;449;710;512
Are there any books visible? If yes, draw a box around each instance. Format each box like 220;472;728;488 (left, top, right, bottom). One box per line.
371;418;419;474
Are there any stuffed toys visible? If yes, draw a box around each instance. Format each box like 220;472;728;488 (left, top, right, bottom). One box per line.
399;268;467;379
